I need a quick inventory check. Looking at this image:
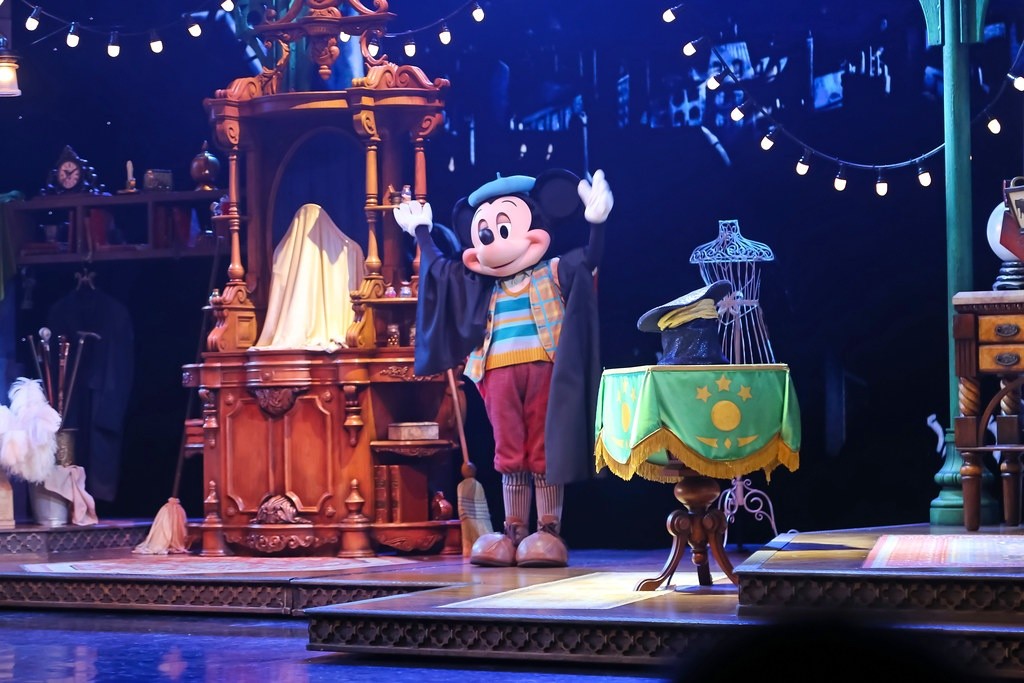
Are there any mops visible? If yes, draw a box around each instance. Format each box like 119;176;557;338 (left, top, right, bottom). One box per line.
128;236;227;556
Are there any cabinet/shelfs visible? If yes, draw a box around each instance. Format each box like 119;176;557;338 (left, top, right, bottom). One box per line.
0;194;244;258
178;0;474;557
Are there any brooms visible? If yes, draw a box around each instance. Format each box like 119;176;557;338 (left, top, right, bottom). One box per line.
446;367;496;564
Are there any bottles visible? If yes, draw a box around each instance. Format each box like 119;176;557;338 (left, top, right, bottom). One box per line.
387;324;400;347
402;185;411;202
409;324;416;346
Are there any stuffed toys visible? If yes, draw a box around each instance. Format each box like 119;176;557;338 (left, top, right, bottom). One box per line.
392;169;615;575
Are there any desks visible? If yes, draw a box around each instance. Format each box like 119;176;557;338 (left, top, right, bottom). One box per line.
952;289;1024;531
592;364;802;592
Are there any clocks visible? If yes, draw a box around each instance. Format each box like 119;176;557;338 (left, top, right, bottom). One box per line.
56;162;81;189
